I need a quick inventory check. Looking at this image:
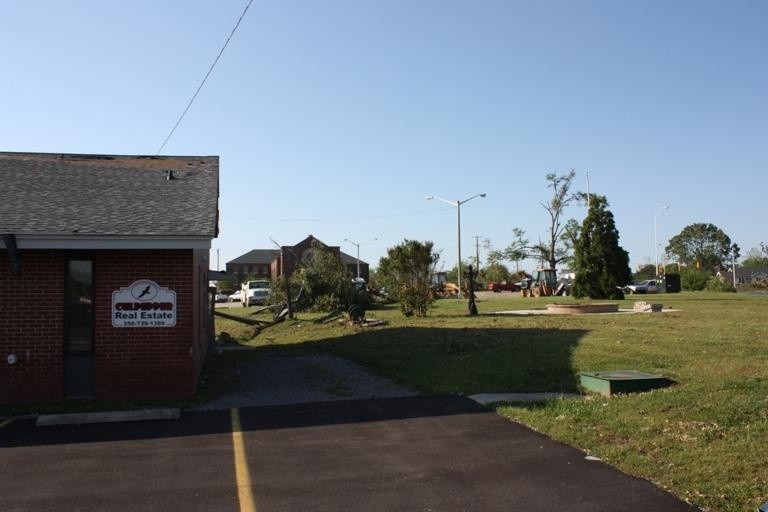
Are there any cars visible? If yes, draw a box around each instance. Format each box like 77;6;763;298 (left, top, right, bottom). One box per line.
214;289;240;303
616;279;657;294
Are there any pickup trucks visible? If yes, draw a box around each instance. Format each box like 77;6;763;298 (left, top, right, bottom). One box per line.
487;278;532;292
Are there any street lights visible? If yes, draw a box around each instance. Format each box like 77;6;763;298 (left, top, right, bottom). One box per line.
342;239;359;286
732;246;736;288
425;193;485;298
267;235;283;277
655;205;669;276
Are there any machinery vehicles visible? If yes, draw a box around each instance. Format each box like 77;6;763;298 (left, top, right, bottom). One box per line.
427;272;476;298
520;269;569;297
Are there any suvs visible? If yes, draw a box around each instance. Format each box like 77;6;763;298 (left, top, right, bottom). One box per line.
240;280;272;307
